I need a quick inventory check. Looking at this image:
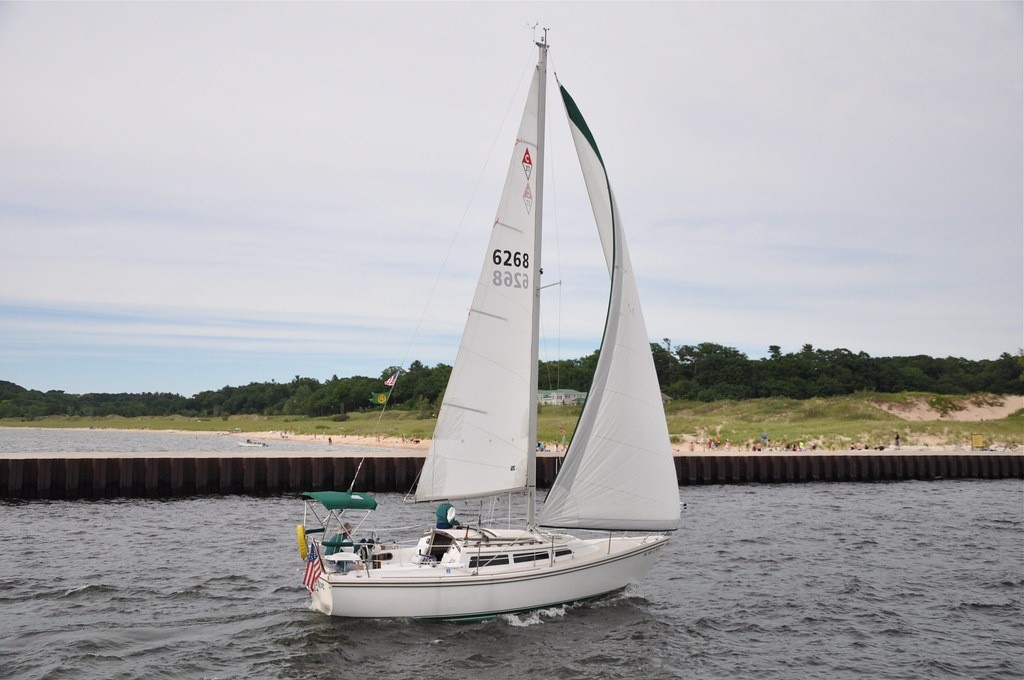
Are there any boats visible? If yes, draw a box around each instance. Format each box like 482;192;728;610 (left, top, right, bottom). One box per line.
236;440;263;448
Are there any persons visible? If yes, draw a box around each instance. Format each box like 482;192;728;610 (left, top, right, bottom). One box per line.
688;430;901;451
436;500;460;529
536;428;567;452
325;523;360;569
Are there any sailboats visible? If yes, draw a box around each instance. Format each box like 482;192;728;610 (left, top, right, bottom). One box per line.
296;15;691;621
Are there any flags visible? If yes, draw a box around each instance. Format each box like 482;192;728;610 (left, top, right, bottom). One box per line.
384;372;398;385
303;544;321;592
369;391;389;405
